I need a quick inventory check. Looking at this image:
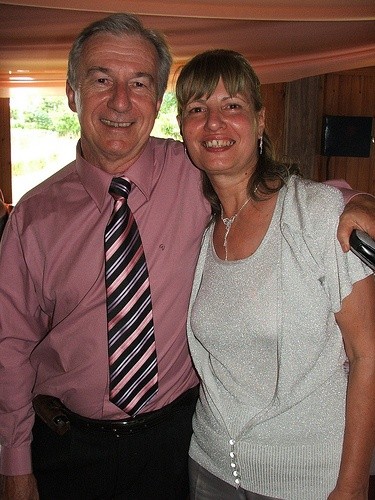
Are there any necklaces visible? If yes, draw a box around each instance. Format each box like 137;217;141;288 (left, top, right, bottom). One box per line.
219;180;263;260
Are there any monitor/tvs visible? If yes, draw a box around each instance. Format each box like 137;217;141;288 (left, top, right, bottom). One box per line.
323;115;372;157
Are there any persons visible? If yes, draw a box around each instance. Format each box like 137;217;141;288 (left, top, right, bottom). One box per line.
0;188;13;240
0;12;374;499
173;47;373;500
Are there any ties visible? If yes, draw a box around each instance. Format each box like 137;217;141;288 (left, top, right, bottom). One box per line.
103;176;159;419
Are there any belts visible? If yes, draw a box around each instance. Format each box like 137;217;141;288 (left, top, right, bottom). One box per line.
56;404;141;441
